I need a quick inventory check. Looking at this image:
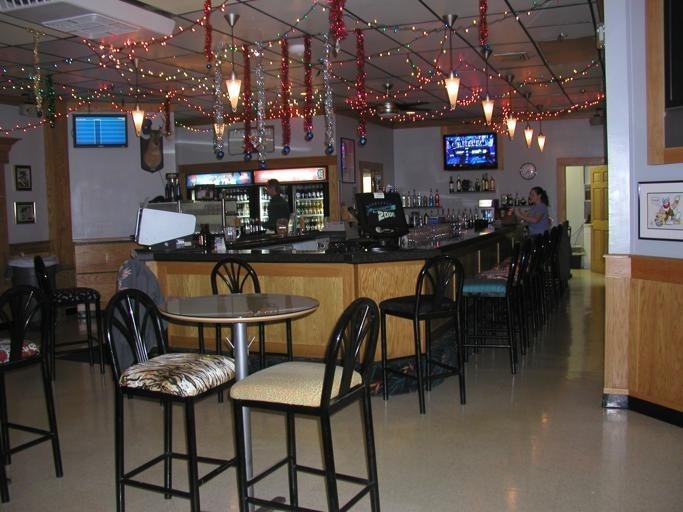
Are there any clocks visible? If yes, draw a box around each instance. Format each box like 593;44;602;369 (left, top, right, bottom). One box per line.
519;163;536;180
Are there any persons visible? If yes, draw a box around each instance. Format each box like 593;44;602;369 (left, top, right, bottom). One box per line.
261;179;290;235
516;187;548;236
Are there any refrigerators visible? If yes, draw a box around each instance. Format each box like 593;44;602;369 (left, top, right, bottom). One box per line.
186;182;330;237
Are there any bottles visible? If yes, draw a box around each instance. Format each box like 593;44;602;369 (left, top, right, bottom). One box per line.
210;187;250;232
258;187;275;231
404;207;482;248
499;192;526;218
401;187;440;207
448;172;495;192
295;186;324;235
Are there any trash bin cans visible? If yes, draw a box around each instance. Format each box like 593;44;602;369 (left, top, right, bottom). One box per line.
7;254;59;330
570;245;584;268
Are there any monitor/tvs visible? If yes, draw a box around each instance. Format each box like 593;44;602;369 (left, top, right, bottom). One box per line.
443;132;498;170
72;113;129;149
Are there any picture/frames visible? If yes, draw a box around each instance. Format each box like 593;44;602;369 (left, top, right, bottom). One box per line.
15;165;32;191
14;201;36;224
638;180;683;242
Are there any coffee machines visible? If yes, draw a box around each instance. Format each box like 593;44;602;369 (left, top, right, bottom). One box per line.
356;193;408;247
164;171;181;203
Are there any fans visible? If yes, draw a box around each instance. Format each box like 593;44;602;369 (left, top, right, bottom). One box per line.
337;83;431;117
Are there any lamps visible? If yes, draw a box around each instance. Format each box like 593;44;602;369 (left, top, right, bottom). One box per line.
225;13;241;111
444;14;546;152
214;123;224;140
131;59;144;138
